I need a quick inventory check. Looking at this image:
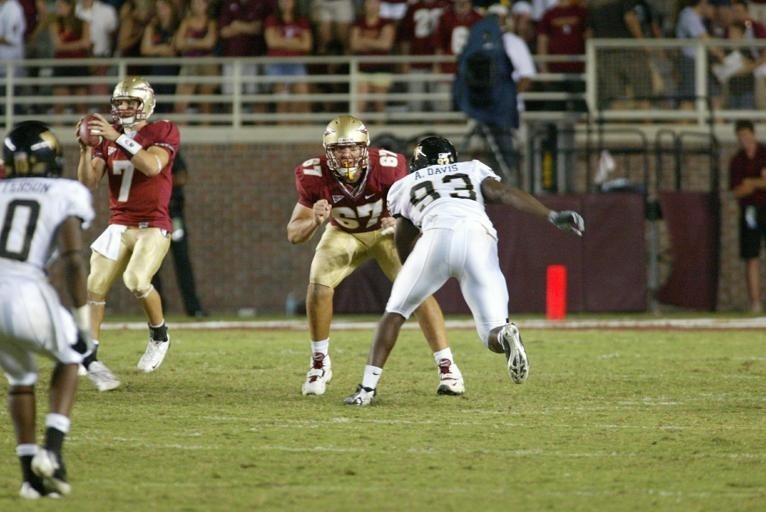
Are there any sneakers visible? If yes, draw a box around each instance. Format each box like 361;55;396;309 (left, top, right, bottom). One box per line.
81;361;119;395
505;324;531;384
16;482;42;498
33;449;68;492
434;358;466;394
138;334;173;375
344;386;377;406
301;353;334;396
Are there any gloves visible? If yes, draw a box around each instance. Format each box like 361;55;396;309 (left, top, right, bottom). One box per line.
549;210;584;235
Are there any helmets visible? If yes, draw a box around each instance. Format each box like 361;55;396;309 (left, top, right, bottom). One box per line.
322;116;371;178
2;121;65;175
115;80;155;132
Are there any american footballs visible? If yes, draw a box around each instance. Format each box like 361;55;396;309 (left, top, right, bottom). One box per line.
78;116;103;146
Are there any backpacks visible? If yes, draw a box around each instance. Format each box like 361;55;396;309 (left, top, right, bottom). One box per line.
466;31;501;112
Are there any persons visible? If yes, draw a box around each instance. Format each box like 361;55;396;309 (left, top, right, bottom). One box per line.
119;0;155;76
350;0;397;125
400;0;451;125
731;0;766;111
0;126;94;497
220;0;266;126
151;151;207;318
50;0;91;126
345;136;585;406
287;115;465;397
264;0;312;125
675;0;725;125
175;0;217;125
77;79;179;374
434;0;482;113
75;0;117;95
487;5;534;191
141;0;180;113
311;43;350;113
723;20;758;110
729;121;766;312
0;0;26;126
313;0;355;55
623;0;674;124
537;0;592;112
19;0;45;114
454;17;516;187
